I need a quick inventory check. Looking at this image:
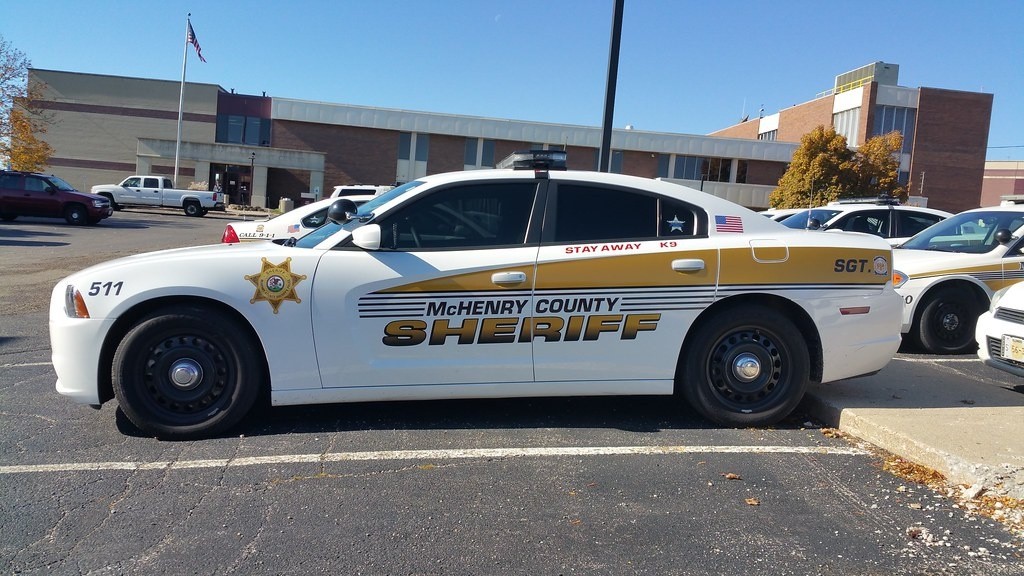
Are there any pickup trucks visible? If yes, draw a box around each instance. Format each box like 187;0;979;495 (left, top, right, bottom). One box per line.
90;176;225;217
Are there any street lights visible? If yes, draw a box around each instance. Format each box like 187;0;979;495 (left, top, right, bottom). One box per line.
700;174;711;191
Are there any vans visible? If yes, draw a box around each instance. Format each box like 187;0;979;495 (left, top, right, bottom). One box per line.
329;183;394;199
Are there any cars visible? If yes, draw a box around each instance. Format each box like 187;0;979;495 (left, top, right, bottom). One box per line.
758;207;928;236
975;281;1024;379
778;196;989;249
220;195;499;250
891;197;1024;355
48;152;905;438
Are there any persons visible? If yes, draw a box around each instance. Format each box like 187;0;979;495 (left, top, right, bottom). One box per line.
213;179;222;192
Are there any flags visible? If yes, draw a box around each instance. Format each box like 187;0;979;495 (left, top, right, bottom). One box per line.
187;21;207;63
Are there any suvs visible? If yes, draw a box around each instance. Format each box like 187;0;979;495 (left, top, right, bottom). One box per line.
0;168;113;226
